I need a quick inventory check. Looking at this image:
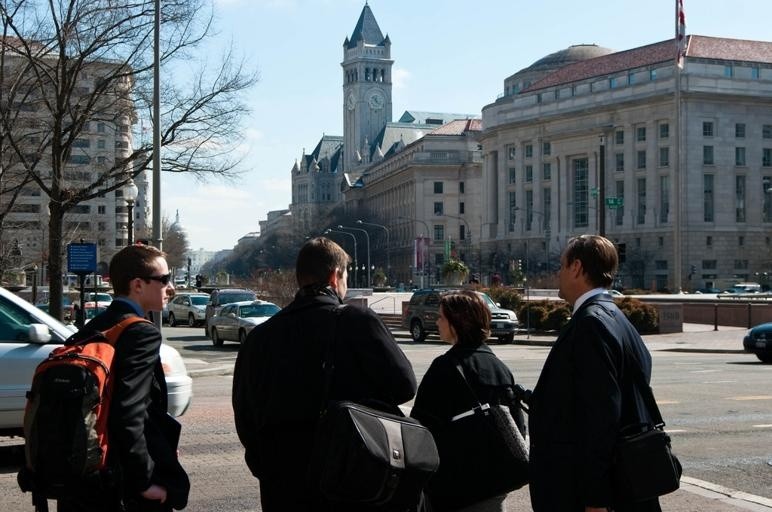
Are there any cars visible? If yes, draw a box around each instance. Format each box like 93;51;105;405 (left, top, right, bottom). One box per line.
743;321;772;364
166;292;208;326
0;286;194;437
207;301;283;347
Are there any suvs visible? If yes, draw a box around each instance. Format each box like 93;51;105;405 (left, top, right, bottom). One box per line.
403;289;520;344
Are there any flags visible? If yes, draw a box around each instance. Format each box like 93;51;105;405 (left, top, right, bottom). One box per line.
676;0;687;70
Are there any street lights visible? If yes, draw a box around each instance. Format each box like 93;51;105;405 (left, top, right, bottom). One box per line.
337;224;371;288
434;211;472;287
397;217;431;287
356;220;390;287
324;228;357;288
513;205;552;287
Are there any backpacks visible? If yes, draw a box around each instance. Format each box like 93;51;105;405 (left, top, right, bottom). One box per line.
17;316;153;512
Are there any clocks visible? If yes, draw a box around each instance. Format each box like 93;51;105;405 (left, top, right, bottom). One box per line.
368;93;384;110
346;95;357;111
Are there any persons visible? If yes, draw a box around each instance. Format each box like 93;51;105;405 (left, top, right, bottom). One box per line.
56;246;190;511
528;236;661;511
410;291;525;511
232;238;416;512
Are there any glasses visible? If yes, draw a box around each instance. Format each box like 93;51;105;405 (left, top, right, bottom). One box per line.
142;272;171;285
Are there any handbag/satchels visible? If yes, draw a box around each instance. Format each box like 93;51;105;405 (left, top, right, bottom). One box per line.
616;421;682;503
423;403;528;512
308;399;441;512
